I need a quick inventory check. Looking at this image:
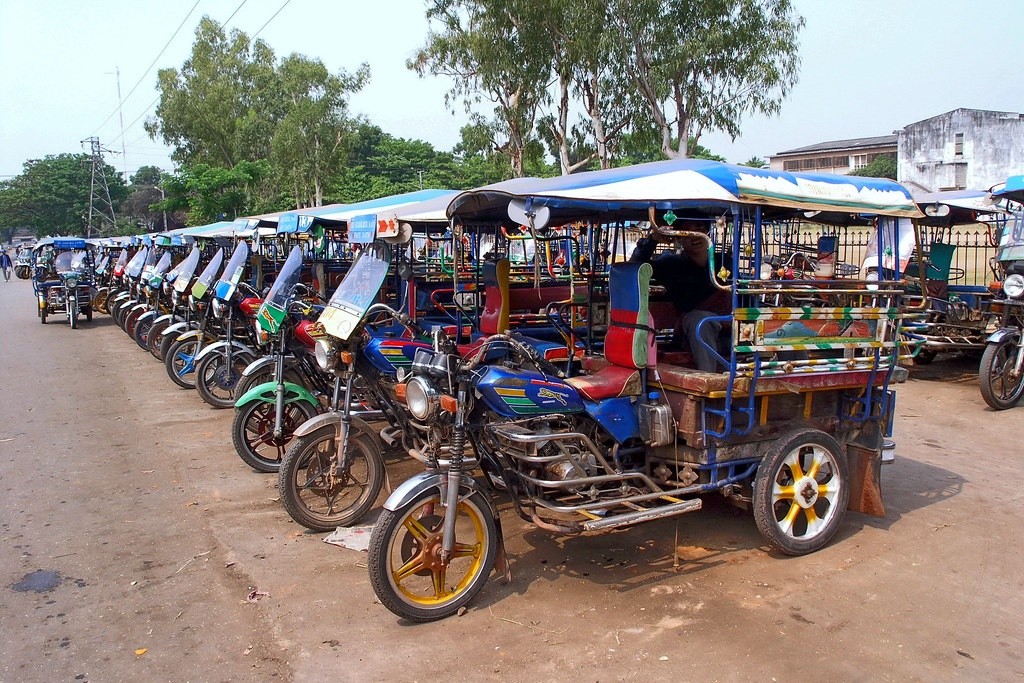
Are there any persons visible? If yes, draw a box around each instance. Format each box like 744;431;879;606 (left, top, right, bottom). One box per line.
628;209;751;373
0;250;14;283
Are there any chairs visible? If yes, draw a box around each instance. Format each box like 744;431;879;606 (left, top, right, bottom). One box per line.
567;260;654;400
456;259;512;361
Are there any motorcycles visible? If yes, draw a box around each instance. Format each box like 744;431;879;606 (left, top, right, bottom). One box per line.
365;161;933;623
30;235;100;330
12;173;1023;534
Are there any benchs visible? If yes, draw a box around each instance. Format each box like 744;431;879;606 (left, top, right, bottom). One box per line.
264;253;924;433
